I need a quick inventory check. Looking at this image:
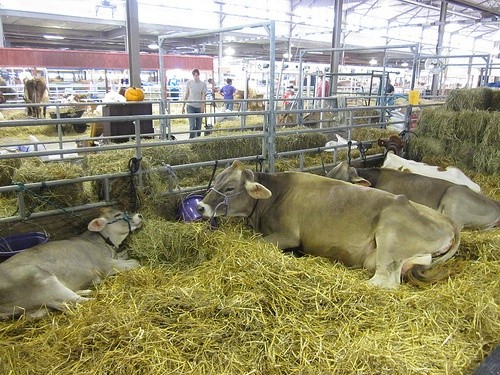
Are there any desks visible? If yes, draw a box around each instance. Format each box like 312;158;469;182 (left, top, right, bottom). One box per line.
103;103;154;144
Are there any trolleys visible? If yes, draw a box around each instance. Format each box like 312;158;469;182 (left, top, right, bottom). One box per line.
49;109;87;135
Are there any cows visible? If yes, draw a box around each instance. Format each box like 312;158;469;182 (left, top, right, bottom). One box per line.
0;207;144;316
196;135;500;289
23;78;47;118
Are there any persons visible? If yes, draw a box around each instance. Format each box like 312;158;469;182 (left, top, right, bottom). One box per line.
385;80;394;115
285;76;331;109
148;74;236;110
182;69;207;138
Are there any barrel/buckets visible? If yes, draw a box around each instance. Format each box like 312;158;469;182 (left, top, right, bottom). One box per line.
409;90;420;104
178;194;217;230
0;219;50;260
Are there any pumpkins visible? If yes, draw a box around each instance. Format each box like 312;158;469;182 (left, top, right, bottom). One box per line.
125;87;144;101
